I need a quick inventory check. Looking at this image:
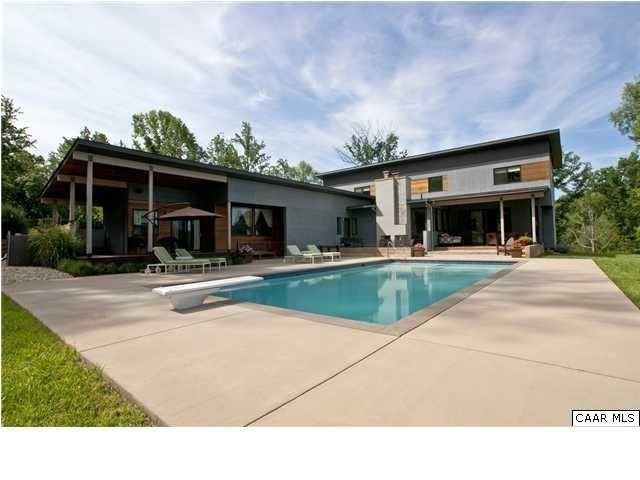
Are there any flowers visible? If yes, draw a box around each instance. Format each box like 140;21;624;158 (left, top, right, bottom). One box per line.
414;243;424;248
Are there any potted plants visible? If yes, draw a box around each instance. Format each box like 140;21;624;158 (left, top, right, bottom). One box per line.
516;236;532;246
510;240;521;258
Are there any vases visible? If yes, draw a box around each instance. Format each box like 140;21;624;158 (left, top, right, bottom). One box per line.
414;247;425;257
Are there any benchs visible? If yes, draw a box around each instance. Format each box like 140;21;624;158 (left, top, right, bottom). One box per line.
238;240;276;260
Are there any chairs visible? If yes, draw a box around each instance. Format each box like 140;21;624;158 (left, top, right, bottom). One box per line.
144;247;226;273
283;244;341;264
497;237;514;256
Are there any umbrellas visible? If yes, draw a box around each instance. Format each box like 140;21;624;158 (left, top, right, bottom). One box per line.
155;207;226;250
